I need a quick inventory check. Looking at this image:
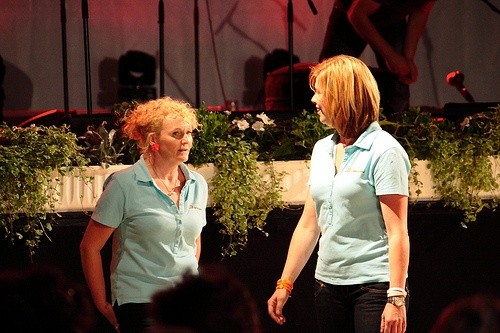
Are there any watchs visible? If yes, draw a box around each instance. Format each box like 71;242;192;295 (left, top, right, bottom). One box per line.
387;297;406;307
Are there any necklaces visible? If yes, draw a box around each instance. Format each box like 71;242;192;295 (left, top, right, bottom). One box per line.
149;155;179;196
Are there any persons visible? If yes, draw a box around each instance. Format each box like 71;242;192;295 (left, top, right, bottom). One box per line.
268;55;411;333
80;96;208;333
148;271;260;333
430;294;500;333
8;280;79;333
319;0;439;84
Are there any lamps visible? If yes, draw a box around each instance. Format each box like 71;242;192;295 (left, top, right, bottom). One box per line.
118;51;156;101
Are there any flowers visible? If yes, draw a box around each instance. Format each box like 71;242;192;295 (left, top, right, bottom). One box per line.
0;102;500;263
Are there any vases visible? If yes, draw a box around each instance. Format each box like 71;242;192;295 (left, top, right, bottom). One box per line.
0;155;500;215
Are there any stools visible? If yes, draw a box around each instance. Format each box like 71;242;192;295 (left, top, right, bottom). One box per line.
264;61;399;111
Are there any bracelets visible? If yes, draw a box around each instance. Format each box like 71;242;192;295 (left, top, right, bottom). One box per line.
276;278;293;294
386;287;408;299
